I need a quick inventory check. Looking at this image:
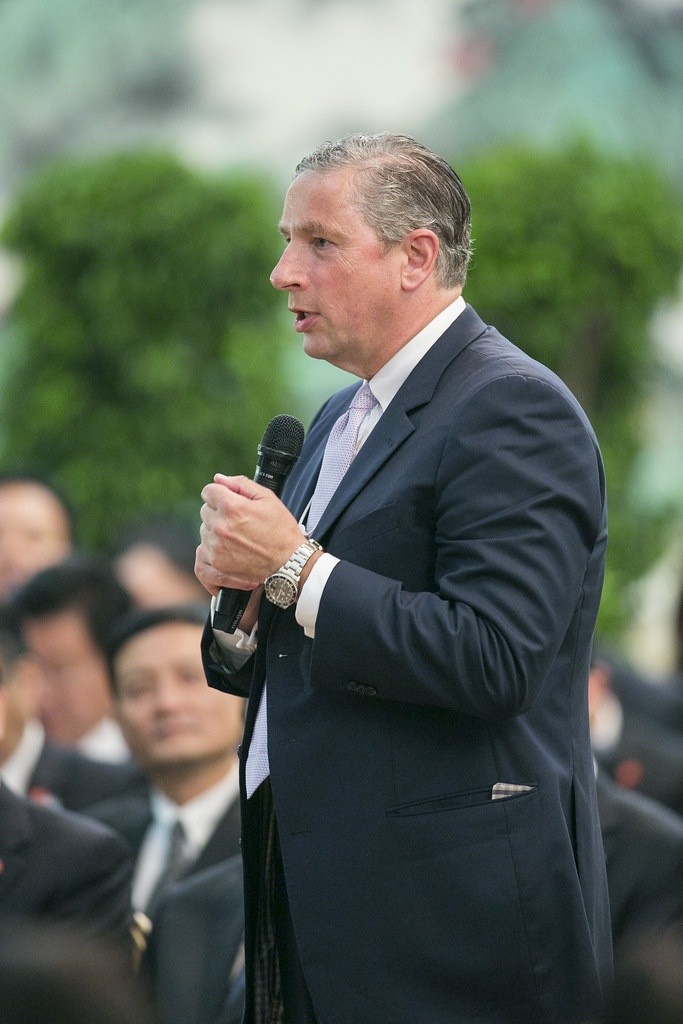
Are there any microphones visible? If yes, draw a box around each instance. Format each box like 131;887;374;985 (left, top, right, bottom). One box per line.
211;415;305;635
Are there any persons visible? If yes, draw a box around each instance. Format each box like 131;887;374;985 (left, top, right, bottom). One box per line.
1;474;683;1024
194;132;611;1023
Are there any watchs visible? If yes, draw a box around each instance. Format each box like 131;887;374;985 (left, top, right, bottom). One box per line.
264;538;323;610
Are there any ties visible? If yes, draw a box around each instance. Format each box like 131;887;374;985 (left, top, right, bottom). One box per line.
244;382;379;801
143;822;187;922
